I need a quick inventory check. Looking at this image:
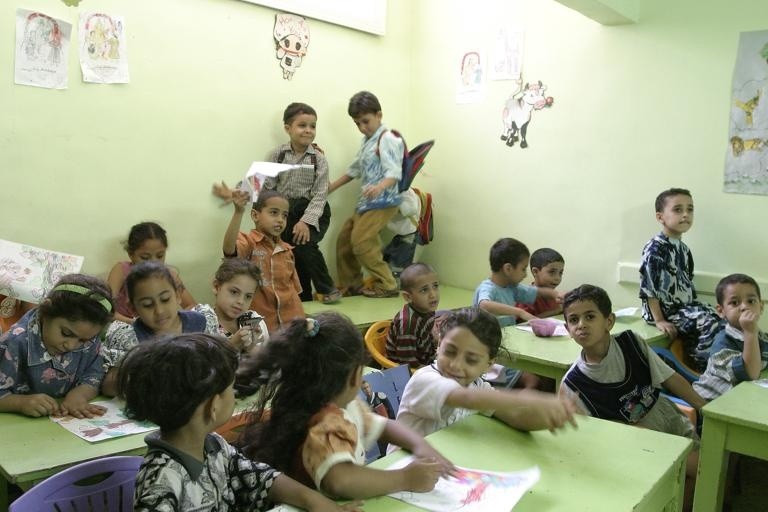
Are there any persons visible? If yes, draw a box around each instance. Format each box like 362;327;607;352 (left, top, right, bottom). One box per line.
357;376;395;453
516;247;564;315
207;259;267;354
211;103;342;303
559;285;707;510
638;186;768;366
224;190;303;328
119;333;368;512
692;273;767;402
386;264;439;370
103;268;218;397
1;273;117;421
381;187;421;273
109;223;195;318
243;313;460;497
386;310;582;455
474;235;565;390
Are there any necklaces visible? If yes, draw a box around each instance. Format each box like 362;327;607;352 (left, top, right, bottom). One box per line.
226;328;238;332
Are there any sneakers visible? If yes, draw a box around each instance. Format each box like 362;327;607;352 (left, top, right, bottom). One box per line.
361;287;399;299
321;290;341;305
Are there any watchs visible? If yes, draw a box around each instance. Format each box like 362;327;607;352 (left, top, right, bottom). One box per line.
327;91;400;299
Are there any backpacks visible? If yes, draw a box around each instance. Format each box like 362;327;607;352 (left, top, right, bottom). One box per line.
407;184;435;244
375;128;434;191
